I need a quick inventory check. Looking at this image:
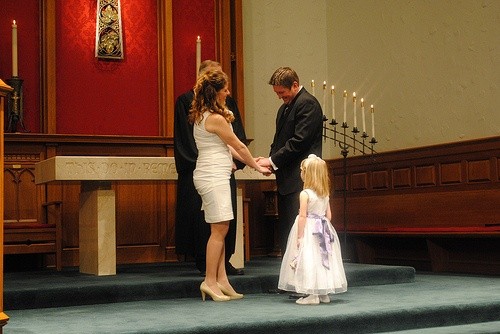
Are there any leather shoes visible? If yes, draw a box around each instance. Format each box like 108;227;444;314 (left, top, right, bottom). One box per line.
197;266;206;276
225;261;244;275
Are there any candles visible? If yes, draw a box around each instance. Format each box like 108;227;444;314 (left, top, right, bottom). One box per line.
311;79;315;96
322;80;327;115
331;84;336;119
361;97;366;132
353;91;357;129
324;116;325;118
12;20;18;78
343;89;347;123
196;34;202;81
371;104;374;138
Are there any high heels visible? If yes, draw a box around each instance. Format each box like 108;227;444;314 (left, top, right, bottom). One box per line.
200;283;230;302
216;281;243;298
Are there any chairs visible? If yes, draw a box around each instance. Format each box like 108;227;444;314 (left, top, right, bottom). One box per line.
3;151;64;272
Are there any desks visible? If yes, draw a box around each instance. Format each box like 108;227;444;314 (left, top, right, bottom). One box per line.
35;156;276;276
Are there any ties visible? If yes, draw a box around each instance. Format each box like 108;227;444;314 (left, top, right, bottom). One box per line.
284;107;289;116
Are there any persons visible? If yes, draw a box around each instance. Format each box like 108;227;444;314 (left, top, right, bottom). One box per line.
188;68;272;302
255;67;323;262
172;59;246;274
277;153;348;305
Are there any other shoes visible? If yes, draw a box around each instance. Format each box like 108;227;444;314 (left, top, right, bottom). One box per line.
295;296;320;304
319;296;330;302
